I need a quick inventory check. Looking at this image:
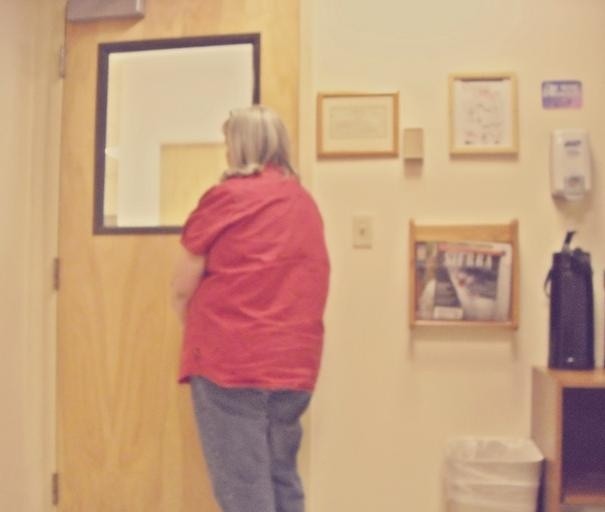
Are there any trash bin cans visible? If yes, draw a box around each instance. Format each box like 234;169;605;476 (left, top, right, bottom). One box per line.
447;437;544;511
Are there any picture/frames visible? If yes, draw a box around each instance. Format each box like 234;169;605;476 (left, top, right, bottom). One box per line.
315;90;400;159
406;218;521;333
447;71;521;155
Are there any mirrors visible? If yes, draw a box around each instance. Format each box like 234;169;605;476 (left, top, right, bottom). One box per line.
91;32;263;236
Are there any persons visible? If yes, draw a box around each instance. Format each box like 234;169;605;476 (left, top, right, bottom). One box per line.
174;105;331;512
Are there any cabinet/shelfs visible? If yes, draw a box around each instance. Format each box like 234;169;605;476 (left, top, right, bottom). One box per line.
530;364;605;511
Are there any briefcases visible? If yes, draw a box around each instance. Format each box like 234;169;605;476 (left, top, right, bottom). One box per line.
551;250;594;370
